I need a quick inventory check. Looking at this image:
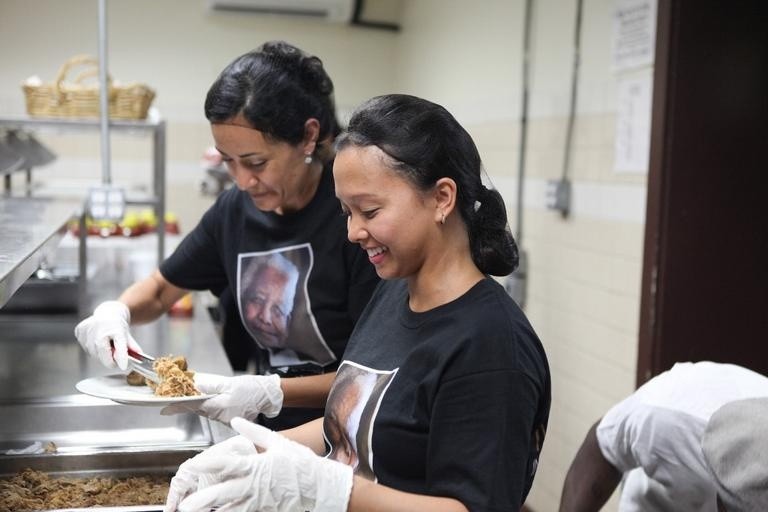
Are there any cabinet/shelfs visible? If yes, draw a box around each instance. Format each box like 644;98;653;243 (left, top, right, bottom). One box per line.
0;115;167;322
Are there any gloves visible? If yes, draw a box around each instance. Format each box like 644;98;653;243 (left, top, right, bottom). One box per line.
164;434;260;512
161;373;284;424
177;416;354;512
74;300;144;371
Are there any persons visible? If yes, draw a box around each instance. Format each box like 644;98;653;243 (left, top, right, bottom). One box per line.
326;365;378;471
559;360;768;512
240;253;300;350
163;94;551;512
73;41;381;432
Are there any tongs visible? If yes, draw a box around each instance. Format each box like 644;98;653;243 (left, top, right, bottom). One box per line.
109;339;164;385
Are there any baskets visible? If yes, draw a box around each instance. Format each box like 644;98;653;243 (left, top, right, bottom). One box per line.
21;56;155;120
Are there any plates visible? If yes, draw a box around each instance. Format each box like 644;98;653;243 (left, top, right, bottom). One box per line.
75;372;218;407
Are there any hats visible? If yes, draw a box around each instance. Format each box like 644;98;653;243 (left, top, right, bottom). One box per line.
700;397;768;510
